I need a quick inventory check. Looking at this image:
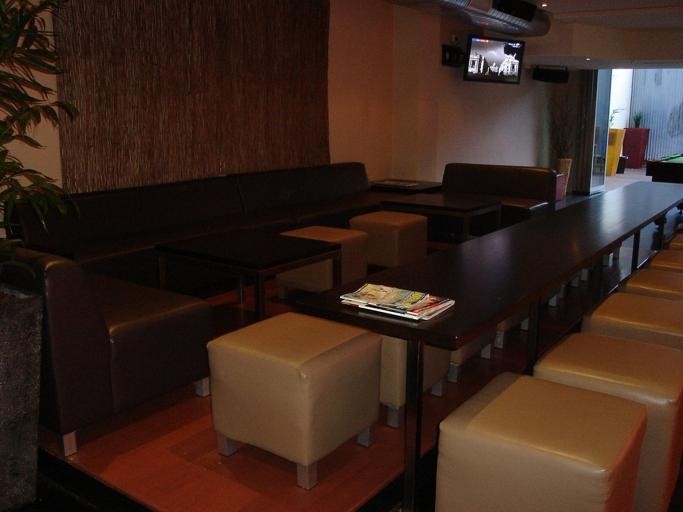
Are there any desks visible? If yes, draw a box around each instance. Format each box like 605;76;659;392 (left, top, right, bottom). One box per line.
646;152;683;183
370;178;442;194
263;180;682;512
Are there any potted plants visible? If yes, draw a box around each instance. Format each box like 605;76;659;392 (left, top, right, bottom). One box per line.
0;2;79;510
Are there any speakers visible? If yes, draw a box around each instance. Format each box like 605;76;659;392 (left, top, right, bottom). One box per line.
533;68;569;84
492;0;537;22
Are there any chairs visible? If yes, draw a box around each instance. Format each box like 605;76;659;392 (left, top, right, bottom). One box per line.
0;240;208;460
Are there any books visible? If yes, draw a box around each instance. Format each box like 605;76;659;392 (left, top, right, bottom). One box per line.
336;282;457;322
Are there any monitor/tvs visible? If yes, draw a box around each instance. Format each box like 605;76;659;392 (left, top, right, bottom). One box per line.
463;33;525;84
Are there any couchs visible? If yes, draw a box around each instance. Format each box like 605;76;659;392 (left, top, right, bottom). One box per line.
5;162;407;286
442;162;558;222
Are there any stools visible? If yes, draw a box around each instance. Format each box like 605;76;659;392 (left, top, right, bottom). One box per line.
206;212;682;512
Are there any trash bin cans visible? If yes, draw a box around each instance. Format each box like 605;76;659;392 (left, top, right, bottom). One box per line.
616;157;626;173
